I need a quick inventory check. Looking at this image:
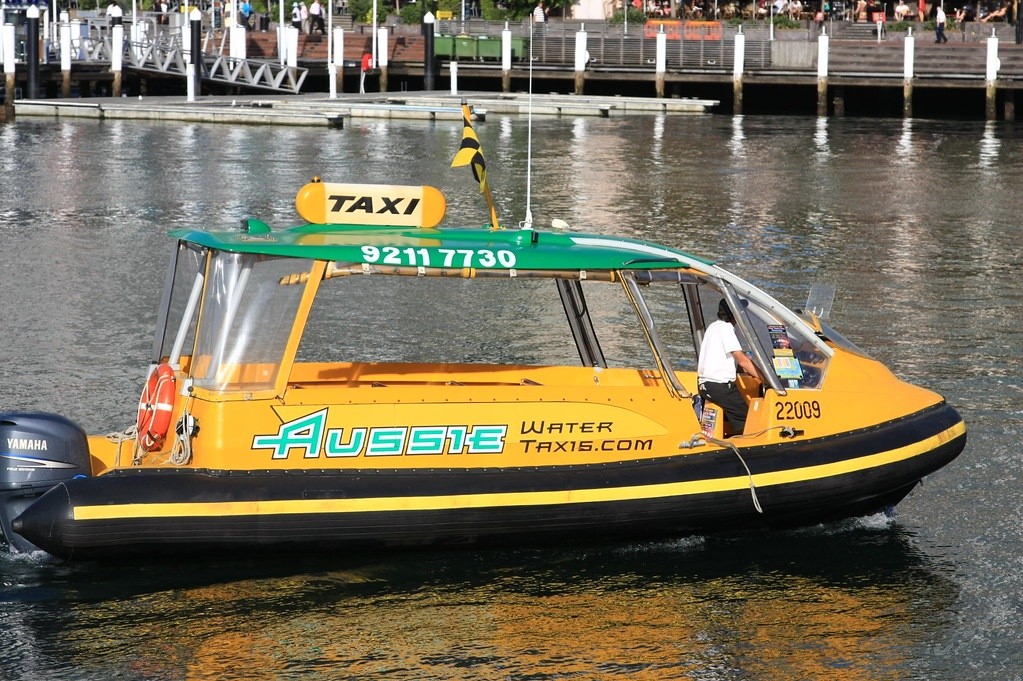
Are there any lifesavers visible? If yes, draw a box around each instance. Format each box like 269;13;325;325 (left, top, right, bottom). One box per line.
135;363;176;452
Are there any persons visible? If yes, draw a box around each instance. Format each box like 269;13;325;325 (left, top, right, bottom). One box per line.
935;7;948;43
532;2;549;36
791;0;801;21
155;0;253;33
335;0;349;15
954;5;974;22
979;1;1011;23
106;1;122;29
895;1;909;21
697;298;768;435
291;0;327;35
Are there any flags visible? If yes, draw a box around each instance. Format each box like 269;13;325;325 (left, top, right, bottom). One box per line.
451;114;487;196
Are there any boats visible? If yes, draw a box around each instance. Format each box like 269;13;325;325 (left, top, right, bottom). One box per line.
0;176;969;562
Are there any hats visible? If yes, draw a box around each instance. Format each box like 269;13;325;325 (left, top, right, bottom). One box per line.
299;2;305;6
293;2;298;6
719;298;748;314
